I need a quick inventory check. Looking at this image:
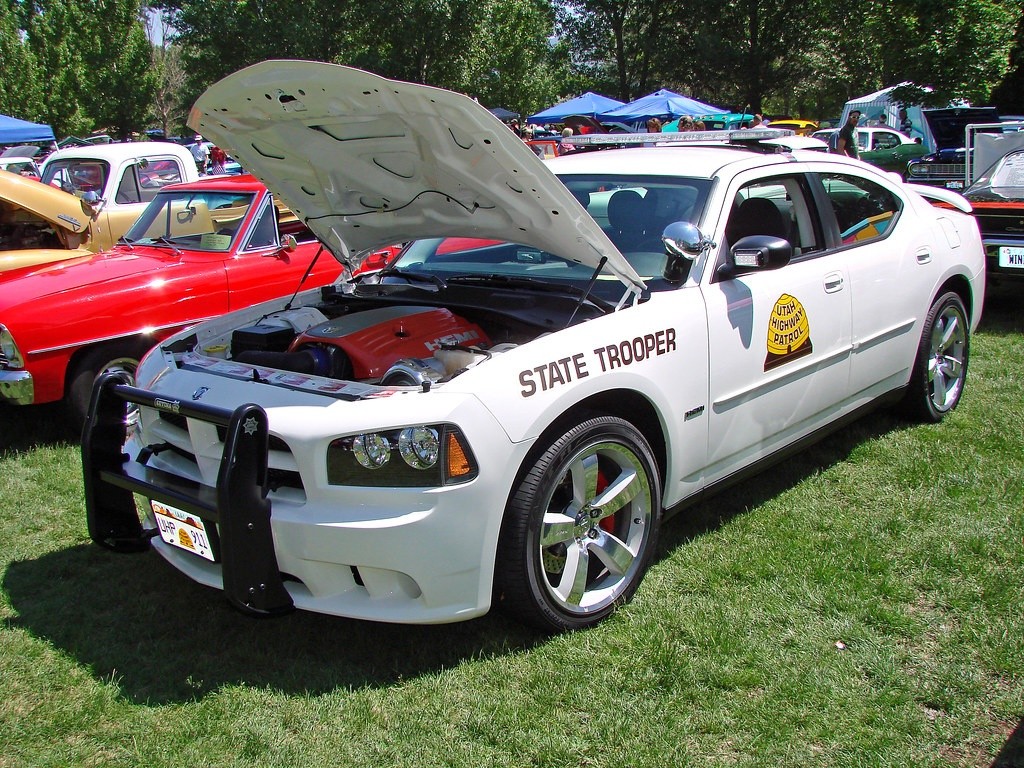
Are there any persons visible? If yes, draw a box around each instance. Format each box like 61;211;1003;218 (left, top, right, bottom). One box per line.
190;134;228;179
503;110;923;160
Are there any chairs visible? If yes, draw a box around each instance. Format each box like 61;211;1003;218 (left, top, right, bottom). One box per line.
725;198;785;247
607;191;648;239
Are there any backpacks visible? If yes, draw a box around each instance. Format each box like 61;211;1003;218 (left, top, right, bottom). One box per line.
829;130;840;150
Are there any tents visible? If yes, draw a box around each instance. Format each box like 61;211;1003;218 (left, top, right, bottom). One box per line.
489;89;733;122
837;80;974;153
0;113;94;153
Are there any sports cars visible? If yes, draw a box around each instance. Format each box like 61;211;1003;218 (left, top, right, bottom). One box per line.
0;171;511;435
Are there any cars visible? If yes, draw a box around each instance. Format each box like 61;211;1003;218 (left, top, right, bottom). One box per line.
0;135;300;272
78;56;987;632
502;104;1024;286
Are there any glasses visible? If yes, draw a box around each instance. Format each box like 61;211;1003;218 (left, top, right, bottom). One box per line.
527;132;532;134
850;115;860;119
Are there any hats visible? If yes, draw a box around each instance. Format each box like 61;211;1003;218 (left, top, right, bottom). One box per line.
195;135;203;141
512;119;517;123
755;114;762;122
849;108;862;116
